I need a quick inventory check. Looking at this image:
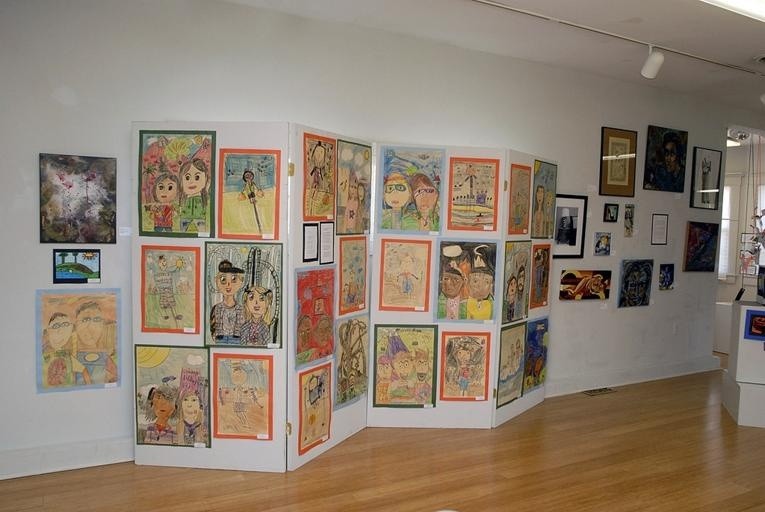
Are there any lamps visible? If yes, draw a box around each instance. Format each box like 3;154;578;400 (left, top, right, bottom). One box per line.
641;42;665;82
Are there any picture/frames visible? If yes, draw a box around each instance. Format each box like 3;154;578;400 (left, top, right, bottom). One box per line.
689;145;723;209
597;126;638;198
552;193;586;259
